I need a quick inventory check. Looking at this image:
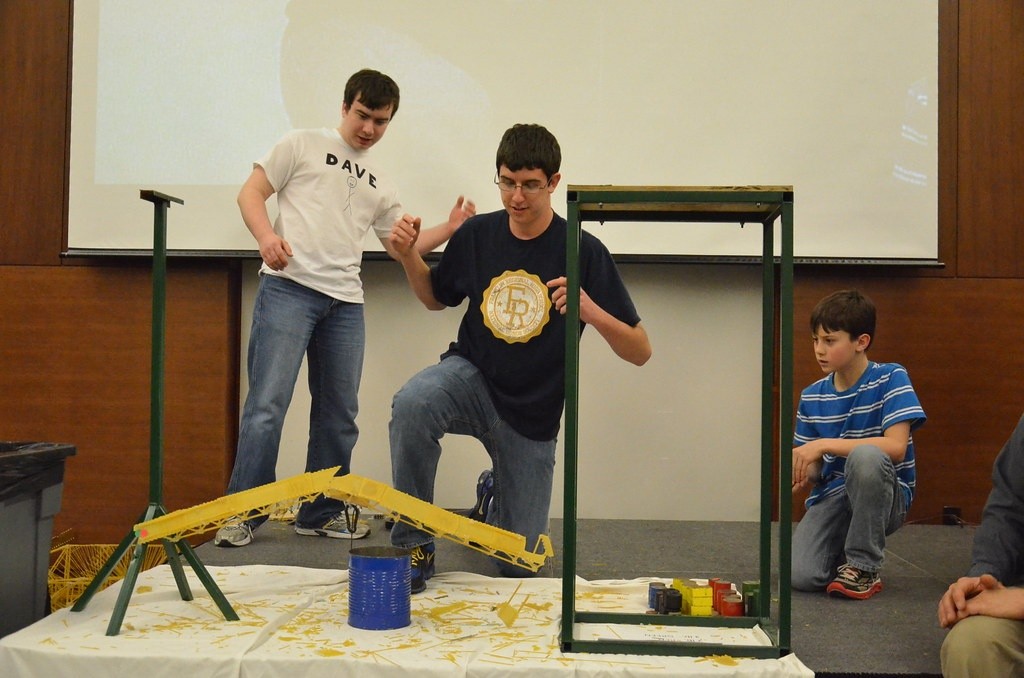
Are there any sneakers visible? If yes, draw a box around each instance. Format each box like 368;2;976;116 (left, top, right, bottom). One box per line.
409;541;435;594
466;469;495;548
826;560;884;600
293;515;371;540
214;515;270;547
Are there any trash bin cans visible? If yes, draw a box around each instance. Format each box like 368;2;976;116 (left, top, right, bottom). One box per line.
0;440;79;646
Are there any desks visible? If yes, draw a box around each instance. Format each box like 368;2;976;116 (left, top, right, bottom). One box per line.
565;183;794;657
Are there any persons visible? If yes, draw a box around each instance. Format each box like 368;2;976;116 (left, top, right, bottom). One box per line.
792;288;926;598
938;412;1024;678
390;124;652;594
215;68;475;547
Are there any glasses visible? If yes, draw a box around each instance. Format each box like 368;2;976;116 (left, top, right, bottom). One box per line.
494;171;554;194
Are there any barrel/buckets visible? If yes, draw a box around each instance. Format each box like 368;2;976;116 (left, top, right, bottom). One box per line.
348;546;411;630
